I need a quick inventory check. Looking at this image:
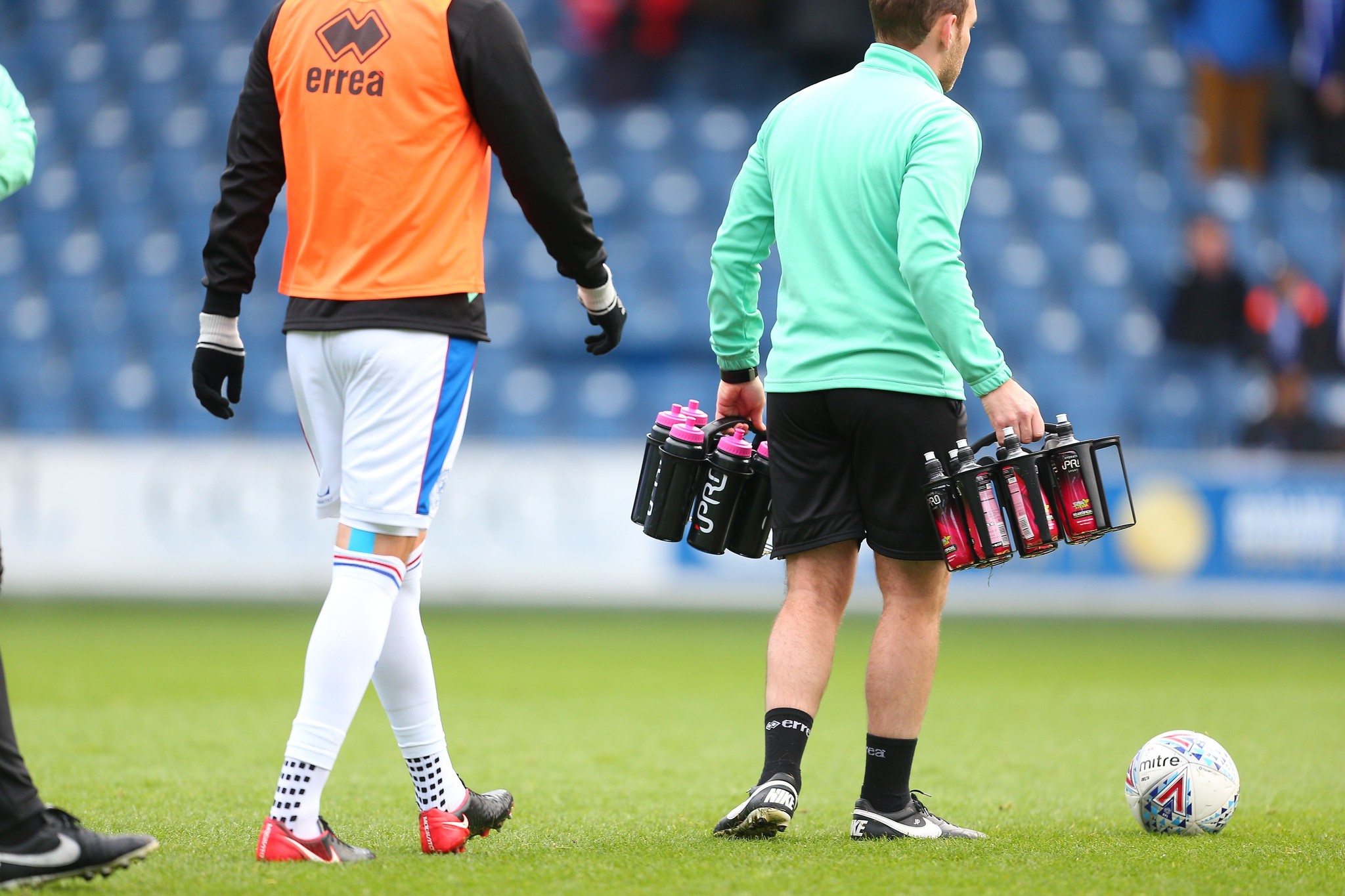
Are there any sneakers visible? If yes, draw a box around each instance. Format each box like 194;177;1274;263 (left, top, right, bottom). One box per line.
257;816;376;864
0;806;159;889
849;788;988;842
713;772;799;839
420;772;515;854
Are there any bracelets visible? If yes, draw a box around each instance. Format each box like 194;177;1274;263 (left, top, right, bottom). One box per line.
718;367;759;383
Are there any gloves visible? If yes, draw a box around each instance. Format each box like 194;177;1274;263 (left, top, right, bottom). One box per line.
578;263;628;355
192;312;246;421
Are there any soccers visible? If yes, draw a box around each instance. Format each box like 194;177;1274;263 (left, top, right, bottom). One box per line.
1124;730;1240;836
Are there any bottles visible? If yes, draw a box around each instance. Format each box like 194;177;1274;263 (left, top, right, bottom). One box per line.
994;426;1060;560
948;439;1016;567
687;427;752;554
1044;411;1106;545
680;399;708;429
924;451;974;571
631;403;686;526
643;416;705;543
725;440;772;559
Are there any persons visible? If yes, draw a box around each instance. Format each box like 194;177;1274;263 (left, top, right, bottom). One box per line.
559;0;872;102
1171;217;1345;452
704;0;1043;835
1159;0;1345;182
189;0;629;861
0;68;160;896
